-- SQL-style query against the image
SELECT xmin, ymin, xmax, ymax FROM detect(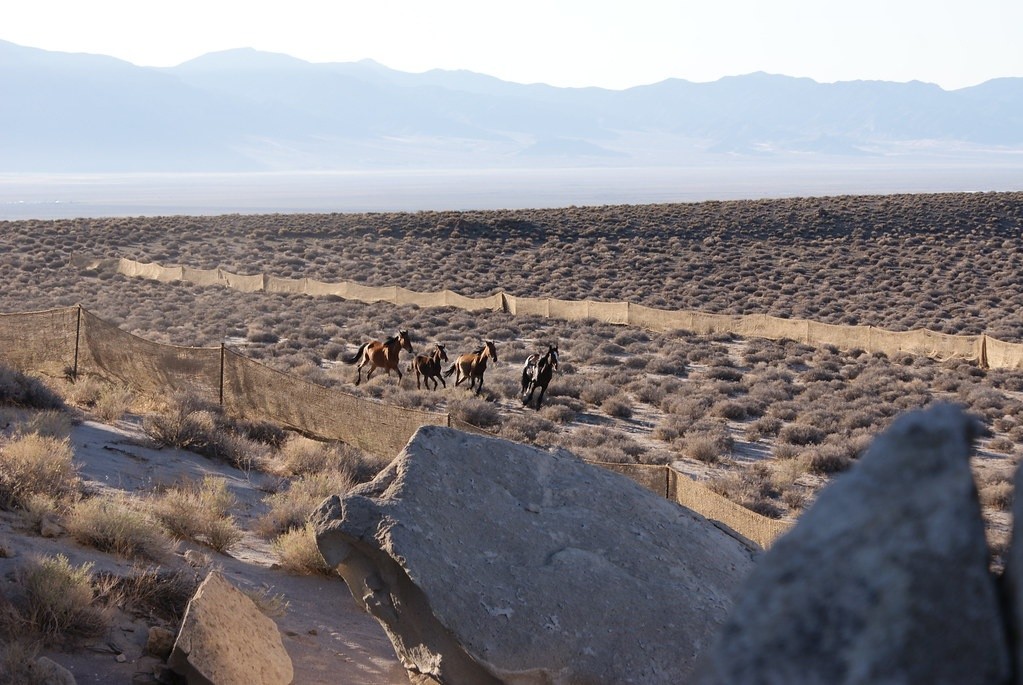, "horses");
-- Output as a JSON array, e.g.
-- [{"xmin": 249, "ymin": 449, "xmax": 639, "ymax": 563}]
[
  {"xmin": 442, "ymin": 339, "xmax": 498, "ymax": 394},
  {"xmin": 521, "ymin": 345, "xmax": 560, "ymax": 412},
  {"xmin": 346, "ymin": 329, "xmax": 412, "ymax": 387},
  {"xmin": 407, "ymin": 344, "xmax": 449, "ymax": 392}
]
